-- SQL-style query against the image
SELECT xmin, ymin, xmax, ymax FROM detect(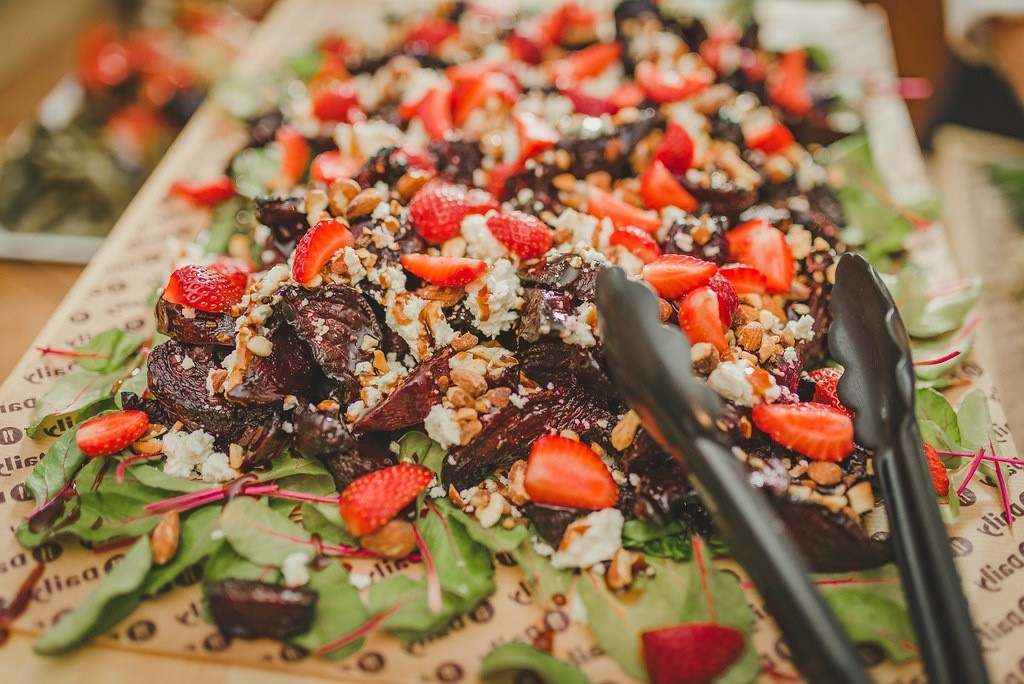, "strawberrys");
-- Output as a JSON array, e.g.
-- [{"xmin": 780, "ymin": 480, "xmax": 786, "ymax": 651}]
[{"xmin": 77, "ymin": 1, "xmax": 950, "ymax": 533}]
